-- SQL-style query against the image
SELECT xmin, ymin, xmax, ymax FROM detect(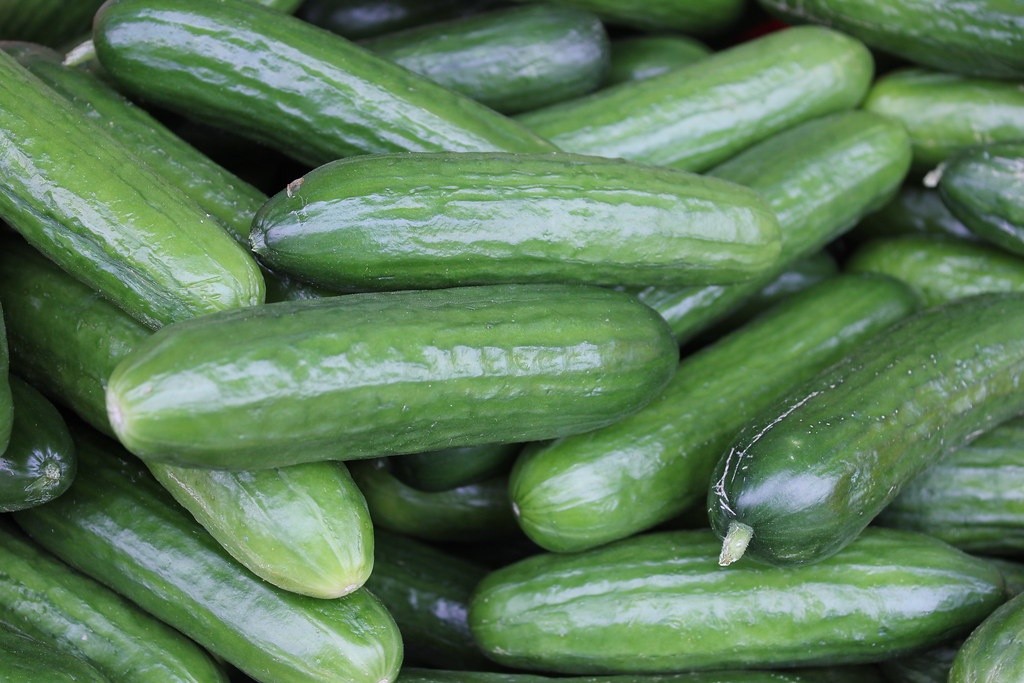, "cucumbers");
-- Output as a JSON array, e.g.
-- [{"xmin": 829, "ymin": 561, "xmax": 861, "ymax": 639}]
[{"xmin": 0, "ymin": 0, "xmax": 1024, "ymax": 683}]
